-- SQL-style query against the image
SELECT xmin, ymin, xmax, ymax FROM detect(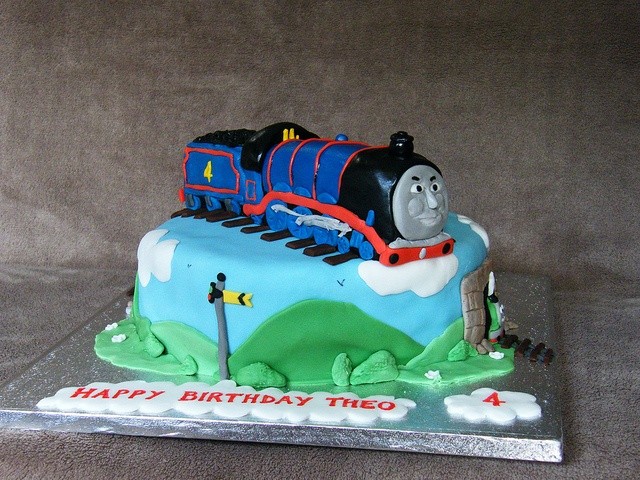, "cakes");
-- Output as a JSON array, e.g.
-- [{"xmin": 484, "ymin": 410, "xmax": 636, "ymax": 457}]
[{"xmin": 35, "ymin": 121, "xmax": 554, "ymax": 424}]
